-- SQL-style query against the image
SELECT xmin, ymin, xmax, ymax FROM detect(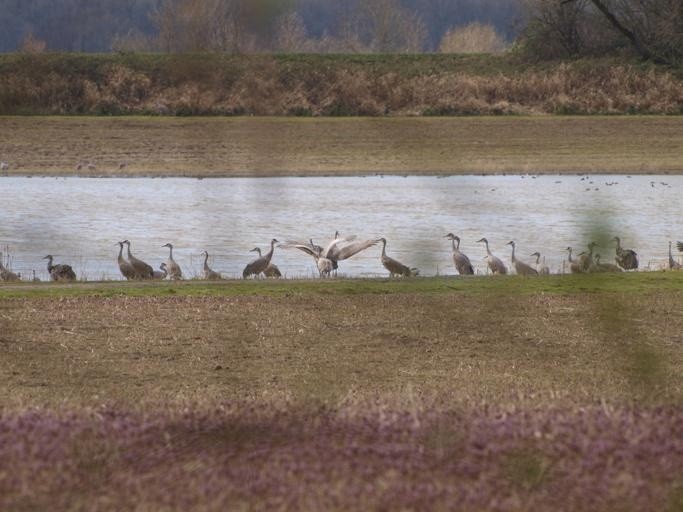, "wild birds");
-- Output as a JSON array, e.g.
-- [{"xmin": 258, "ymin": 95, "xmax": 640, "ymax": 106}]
[
  {"xmin": 41, "ymin": 255, "xmax": 76, "ymax": 282},
  {"xmin": 668, "ymin": 241, "xmax": 682, "ymax": 271},
  {"xmin": 112, "ymin": 238, "xmax": 183, "ymax": 281},
  {"xmin": 442, "ymin": 232, "xmax": 549, "ymax": 275},
  {"xmin": 564, "ymin": 234, "xmax": 638, "ymax": 274},
  {"xmin": 241, "ymin": 229, "xmax": 418, "ymax": 278},
  {"xmin": 198, "ymin": 250, "xmax": 222, "ymax": 282}
]
